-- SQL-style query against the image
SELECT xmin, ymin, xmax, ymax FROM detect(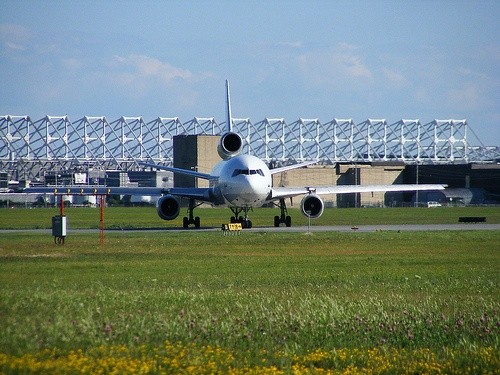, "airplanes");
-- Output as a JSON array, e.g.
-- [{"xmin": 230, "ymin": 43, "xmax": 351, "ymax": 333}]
[{"xmin": 24, "ymin": 78, "xmax": 449, "ymax": 229}]
